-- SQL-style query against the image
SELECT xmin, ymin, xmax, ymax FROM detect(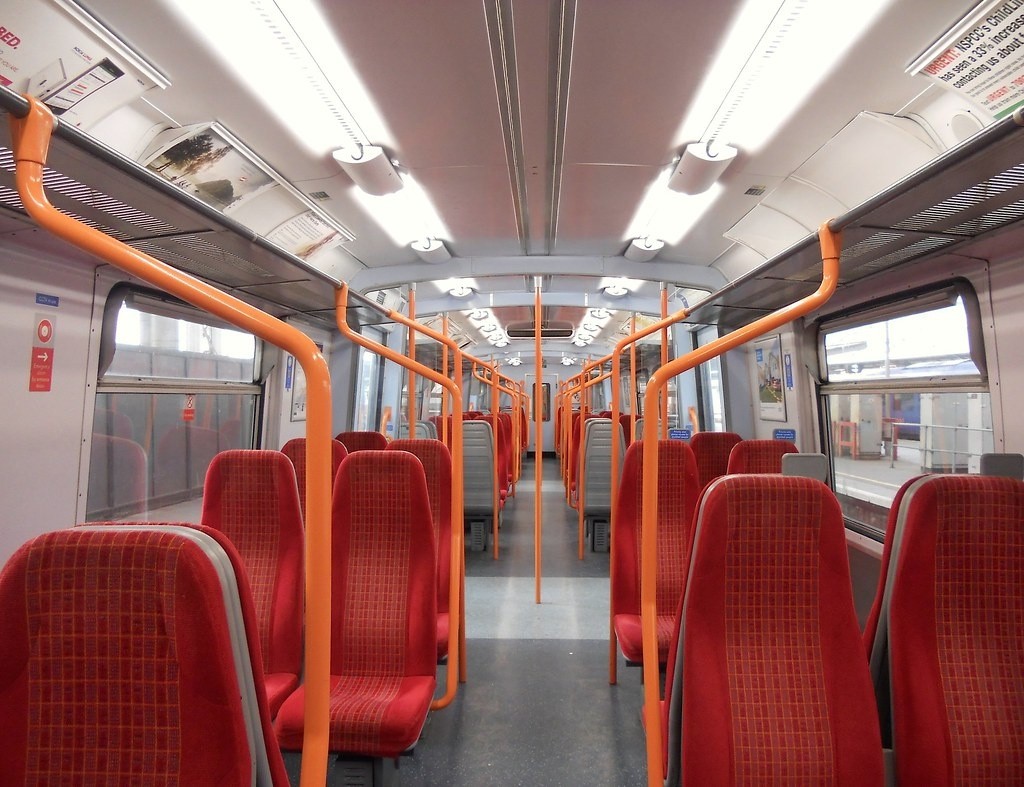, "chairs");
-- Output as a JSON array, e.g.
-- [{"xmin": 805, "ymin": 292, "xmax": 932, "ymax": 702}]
[
  {"xmin": 641, "ymin": 474, "xmax": 887, "ymax": 786},
  {"xmin": 0, "ymin": 521, "xmax": 290, "ymax": 787},
  {"xmin": 613, "ymin": 439, "xmax": 700, "ymax": 682},
  {"xmin": 863, "ymin": 474, "xmax": 1024, "ymax": 787},
  {"xmin": 271, "ymin": 450, "xmax": 437, "ymax": 787},
  {"xmin": 280, "ymin": 438, "xmax": 348, "ymax": 529},
  {"xmin": 554, "ymin": 406, "xmax": 662, "ymax": 553},
  {"xmin": 384, "ymin": 439, "xmax": 453, "ymax": 665},
  {"xmin": 689, "ymin": 432, "xmax": 742, "ymax": 491},
  {"xmin": 199, "ymin": 449, "xmax": 305, "ymax": 722},
  {"xmin": 398, "ymin": 407, "xmax": 527, "ymax": 553},
  {"xmin": 726, "ymin": 440, "xmax": 799, "ymax": 474},
  {"xmin": 334, "ymin": 432, "xmax": 388, "ymax": 454}
]
